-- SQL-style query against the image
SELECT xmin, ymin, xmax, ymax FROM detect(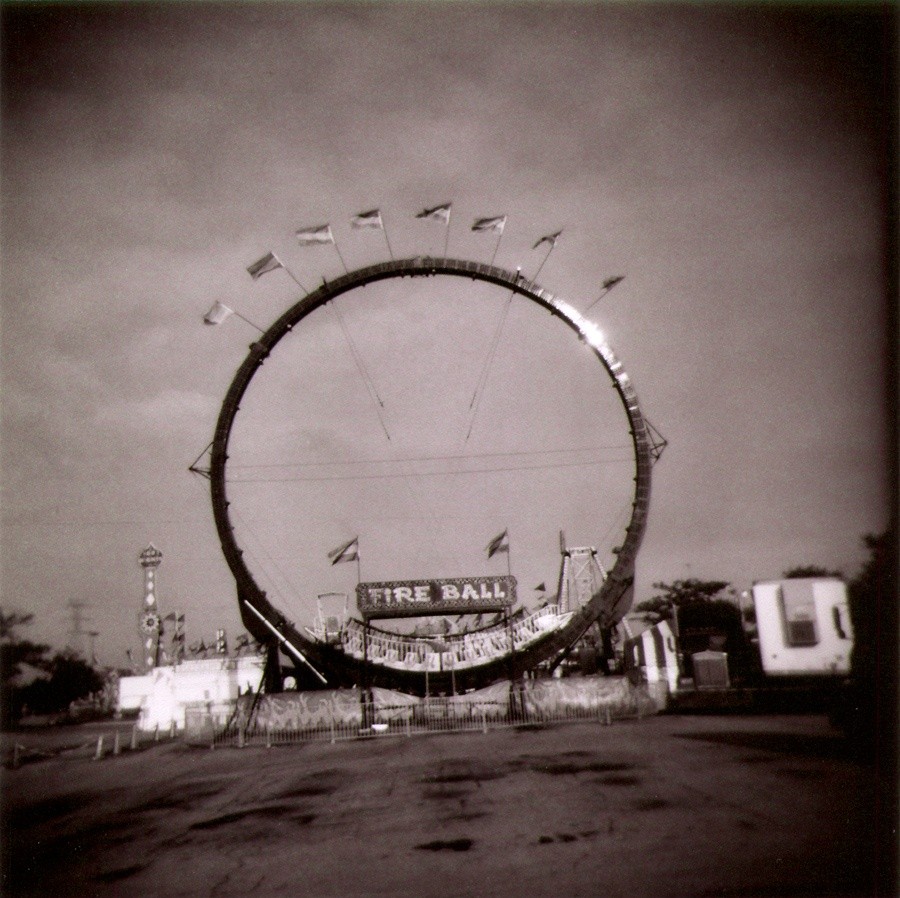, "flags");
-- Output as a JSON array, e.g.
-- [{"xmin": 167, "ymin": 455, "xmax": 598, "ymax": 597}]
[
  {"xmin": 484, "ymin": 529, "xmax": 510, "ymax": 559},
  {"xmin": 414, "ymin": 201, "xmax": 452, "ymax": 225},
  {"xmin": 532, "ymin": 228, "xmax": 563, "ymax": 250},
  {"xmin": 177, "ymin": 614, "xmax": 186, "ymax": 623},
  {"xmin": 534, "ymin": 583, "xmax": 546, "ymax": 593},
  {"xmin": 247, "ymin": 253, "xmax": 285, "ymax": 281},
  {"xmin": 295, "ymin": 222, "xmax": 335, "ymax": 248},
  {"xmin": 472, "ymin": 215, "xmax": 507, "ymax": 235},
  {"xmin": 203, "ymin": 302, "xmax": 232, "ymax": 325},
  {"xmin": 163, "ymin": 612, "xmax": 177, "ymax": 623},
  {"xmin": 328, "ymin": 536, "xmax": 361, "ymax": 566},
  {"xmin": 349, "ymin": 207, "xmax": 384, "ymax": 231},
  {"xmin": 602, "ymin": 274, "xmax": 625, "ymax": 292}
]
